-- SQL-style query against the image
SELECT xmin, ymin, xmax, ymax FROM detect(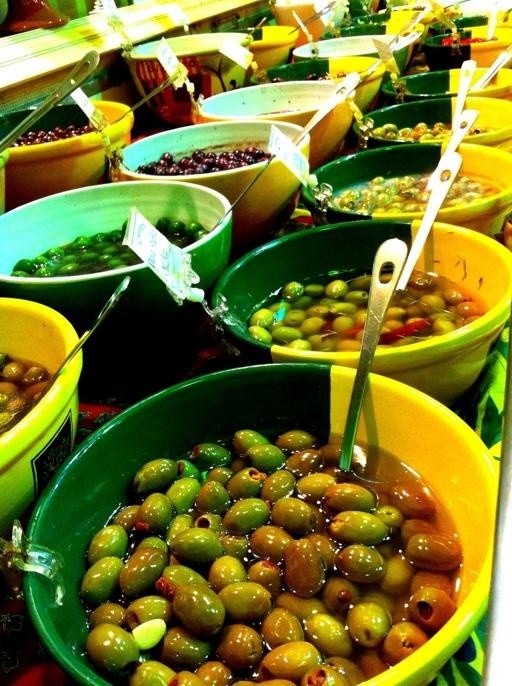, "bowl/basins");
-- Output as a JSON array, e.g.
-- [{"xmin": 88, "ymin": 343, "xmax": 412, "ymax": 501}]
[
  {"xmin": 1, "ymin": 0, "xmax": 511, "ymax": 172},
  {"xmin": 112, "ymin": 117, "xmax": 312, "ymax": 269},
  {"xmin": 0, "ymin": 94, "xmax": 139, "ymax": 215},
  {"xmin": 1, "ymin": 297, "xmax": 86, "ymax": 535},
  {"xmin": 300, "ymin": 139, "xmax": 512, "ymax": 245},
  {"xmin": 0, "ymin": 182, "xmax": 235, "ymax": 354},
  {"xmin": 19, "ymin": 361, "xmax": 512, "ymax": 686},
  {"xmin": 208, "ymin": 220, "xmax": 510, "ymax": 406}
]
